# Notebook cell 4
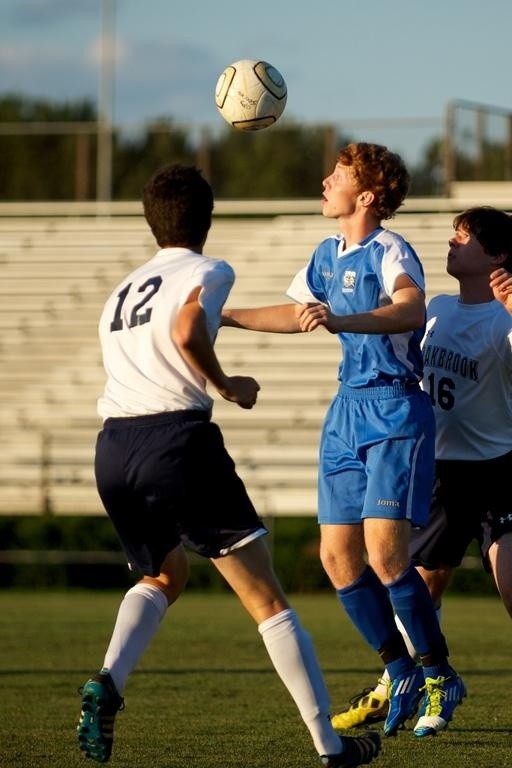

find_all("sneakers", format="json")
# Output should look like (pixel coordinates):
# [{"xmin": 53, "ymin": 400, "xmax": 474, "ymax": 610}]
[
  {"xmin": 321, "ymin": 733, "xmax": 381, "ymax": 768},
  {"xmin": 332, "ymin": 678, "xmax": 390, "ymax": 728},
  {"xmin": 384, "ymin": 667, "xmax": 425, "ymax": 735},
  {"xmin": 413, "ymin": 673, "xmax": 467, "ymax": 736},
  {"xmin": 76, "ymin": 669, "xmax": 123, "ymax": 762}
]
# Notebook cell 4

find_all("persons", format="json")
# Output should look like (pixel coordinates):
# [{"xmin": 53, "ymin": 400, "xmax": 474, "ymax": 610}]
[
  {"xmin": 326, "ymin": 204, "xmax": 512, "ymax": 729},
  {"xmin": 76, "ymin": 163, "xmax": 381, "ymax": 766},
  {"xmin": 213, "ymin": 140, "xmax": 468, "ymax": 737}
]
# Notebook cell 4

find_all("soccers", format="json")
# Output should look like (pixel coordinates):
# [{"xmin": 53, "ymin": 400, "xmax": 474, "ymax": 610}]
[{"xmin": 214, "ymin": 59, "xmax": 286, "ymax": 131}]
